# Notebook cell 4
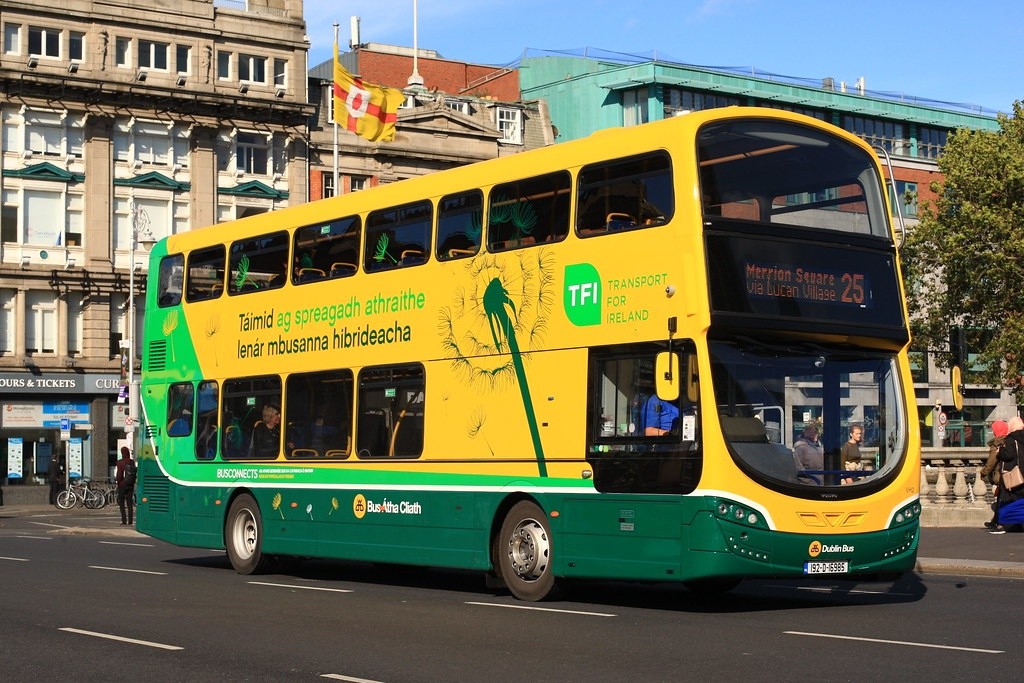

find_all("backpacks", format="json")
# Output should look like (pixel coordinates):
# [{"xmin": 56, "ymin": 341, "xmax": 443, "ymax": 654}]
[{"xmin": 121, "ymin": 459, "xmax": 138, "ymax": 485}]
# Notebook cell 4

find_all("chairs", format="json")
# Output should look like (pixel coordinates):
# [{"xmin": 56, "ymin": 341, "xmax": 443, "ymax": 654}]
[
  {"xmin": 158, "ymin": 213, "xmax": 665, "ymax": 306},
  {"xmin": 628, "ymin": 394, "xmax": 648, "ymax": 451},
  {"xmin": 167, "ymin": 407, "xmax": 417, "ymax": 460}
]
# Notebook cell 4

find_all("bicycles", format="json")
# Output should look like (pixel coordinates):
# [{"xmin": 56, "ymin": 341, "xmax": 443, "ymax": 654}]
[
  {"xmin": 57, "ymin": 477, "xmax": 106, "ymax": 509},
  {"xmin": 80, "ymin": 476, "xmax": 136, "ymax": 510}
]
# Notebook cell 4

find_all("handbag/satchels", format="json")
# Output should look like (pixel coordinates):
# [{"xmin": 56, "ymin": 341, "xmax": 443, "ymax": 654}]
[{"xmin": 1002, "ymin": 466, "xmax": 1024, "ymax": 489}]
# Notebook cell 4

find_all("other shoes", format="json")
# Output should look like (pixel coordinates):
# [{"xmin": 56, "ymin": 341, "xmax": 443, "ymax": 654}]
[
  {"xmin": 990, "ymin": 527, "xmax": 1006, "ymax": 534},
  {"xmin": 985, "ymin": 522, "xmax": 996, "ymax": 529}
]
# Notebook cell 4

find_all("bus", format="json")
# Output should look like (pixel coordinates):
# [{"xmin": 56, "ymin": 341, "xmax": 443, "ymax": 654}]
[{"xmin": 131, "ymin": 104, "xmax": 966, "ymax": 603}]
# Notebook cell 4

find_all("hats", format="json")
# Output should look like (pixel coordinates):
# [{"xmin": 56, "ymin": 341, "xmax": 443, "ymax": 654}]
[
  {"xmin": 1007, "ymin": 417, "xmax": 1024, "ymax": 432},
  {"xmin": 991, "ymin": 421, "xmax": 1009, "ymax": 436}
]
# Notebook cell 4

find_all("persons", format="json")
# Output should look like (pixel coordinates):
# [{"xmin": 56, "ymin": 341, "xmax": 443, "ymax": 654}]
[
  {"xmin": 268, "ymin": 254, "xmax": 303, "ymax": 286},
  {"xmin": 47, "ymin": 454, "xmax": 65, "ymax": 505},
  {"xmin": 254, "ymin": 402, "xmax": 300, "ymax": 460},
  {"xmin": 793, "ymin": 425, "xmax": 827, "ymax": 486},
  {"xmin": 980, "ymin": 417, "xmax": 1024, "ymax": 534},
  {"xmin": 841, "ymin": 424, "xmax": 866, "ymax": 484},
  {"xmin": 114, "ymin": 447, "xmax": 138, "ymax": 526},
  {"xmin": 639, "ymin": 394, "xmax": 678, "ymax": 436}
]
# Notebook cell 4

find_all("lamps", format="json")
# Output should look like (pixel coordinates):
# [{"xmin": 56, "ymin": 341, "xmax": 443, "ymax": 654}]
[
  {"xmin": 274, "ymin": 88, "xmax": 286, "ymax": 100},
  {"xmin": 65, "ymin": 154, "xmax": 75, "ymax": 170},
  {"xmin": 133, "ymin": 262, "xmax": 143, "ymax": 273},
  {"xmin": 66, "ymin": 359, "xmax": 78, "ymax": 367},
  {"xmin": 238, "ymin": 84, "xmax": 250, "ymax": 96},
  {"xmin": 19, "ymin": 256, "xmax": 32, "ymax": 270},
  {"xmin": 172, "ymin": 163, "xmax": 182, "ymax": 180},
  {"xmin": 176, "ymin": 76, "xmax": 186, "ymax": 89},
  {"xmin": 68, "ymin": 63, "xmax": 79, "ymax": 73},
  {"xmin": 64, "ymin": 258, "xmax": 76, "ymax": 271},
  {"xmin": 133, "ymin": 159, "xmax": 143, "ymax": 173},
  {"xmin": 27, "ymin": 58, "xmax": 39, "ymax": 71},
  {"xmin": 234, "ymin": 169, "xmax": 244, "ymax": 184},
  {"xmin": 20, "ymin": 149, "xmax": 33, "ymax": 166},
  {"xmin": 137, "ymin": 72, "xmax": 148, "ymax": 81},
  {"xmin": 272, "ymin": 172, "xmax": 282, "ymax": 188},
  {"xmin": 23, "ymin": 357, "xmax": 34, "ymax": 367}
]
